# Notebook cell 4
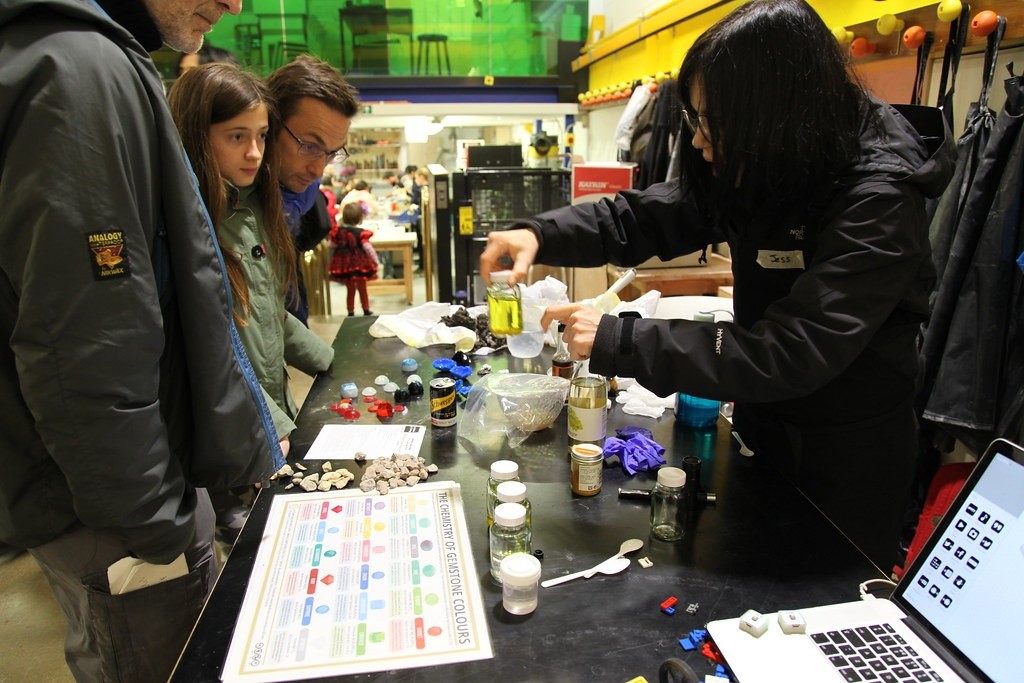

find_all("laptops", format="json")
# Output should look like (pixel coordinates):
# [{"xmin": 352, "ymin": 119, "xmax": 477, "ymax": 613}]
[{"xmin": 703, "ymin": 437, "xmax": 1024, "ymax": 683}]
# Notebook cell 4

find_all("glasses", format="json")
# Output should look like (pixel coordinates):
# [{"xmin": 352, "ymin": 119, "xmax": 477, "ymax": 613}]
[
  {"xmin": 681, "ymin": 109, "xmax": 713, "ymax": 146},
  {"xmin": 282, "ymin": 123, "xmax": 349, "ymax": 165}
]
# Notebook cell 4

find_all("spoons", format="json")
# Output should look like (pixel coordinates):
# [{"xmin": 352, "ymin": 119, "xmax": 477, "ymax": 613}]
[
  {"xmin": 731, "ymin": 431, "xmax": 754, "ymax": 456},
  {"xmin": 584, "ymin": 538, "xmax": 644, "ymax": 579},
  {"xmin": 541, "ymin": 559, "xmax": 631, "ymax": 587}
]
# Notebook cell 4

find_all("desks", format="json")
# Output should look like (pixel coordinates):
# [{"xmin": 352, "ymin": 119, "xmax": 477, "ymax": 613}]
[
  {"xmin": 606, "ymin": 254, "xmax": 734, "ymax": 302},
  {"xmin": 166, "ymin": 316, "xmax": 897, "ymax": 683},
  {"xmin": 365, "ymin": 231, "xmax": 418, "ymax": 307},
  {"xmin": 338, "ymin": 8, "xmax": 414, "ymax": 76}
]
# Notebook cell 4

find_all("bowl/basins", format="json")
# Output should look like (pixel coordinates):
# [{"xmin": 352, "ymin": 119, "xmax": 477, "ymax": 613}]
[
  {"xmin": 489, "ymin": 373, "xmax": 571, "ymax": 432},
  {"xmin": 507, "ymin": 321, "xmax": 544, "ymax": 357}
]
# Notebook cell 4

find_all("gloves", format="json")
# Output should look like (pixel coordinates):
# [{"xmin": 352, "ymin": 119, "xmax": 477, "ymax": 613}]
[{"xmin": 602, "ymin": 426, "xmax": 667, "ymax": 476}]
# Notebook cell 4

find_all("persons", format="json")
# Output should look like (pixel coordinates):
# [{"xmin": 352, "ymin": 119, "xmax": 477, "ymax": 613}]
[
  {"xmin": 479, "ymin": 0, "xmax": 960, "ymax": 581},
  {"xmin": 321, "ymin": 174, "xmax": 338, "ymax": 227},
  {"xmin": 382, "ymin": 164, "xmax": 429, "ymax": 277},
  {"xmin": 341, "ymin": 181, "xmax": 374, "ymax": 206},
  {"xmin": 167, "ymin": 45, "xmax": 358, "ymax": 545},
  {"xmin": 328, "ymin": 203, "xmax": 380, "ymax": 316},
  {"xmin": 0, "ymin": 0, "xmax": 288, "ymax": 683}
]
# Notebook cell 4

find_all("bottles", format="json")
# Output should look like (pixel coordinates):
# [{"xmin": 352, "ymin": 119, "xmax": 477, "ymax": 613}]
[
  {"xmin": 570, "ymin": 444, "xmax": 604, "ymax": 496},
  {"xmin": 650, "ymin": 467, "xmax": 687, "ymax": 543},
  {"xmin": 487, "ymin": 271, "xmax": 522, "ymax": 336},
  {"xmin": 499, "ymin": 552, "xmax": 541, "ymax": 615},
  {"xmin": 567, "ymin": 358, "xmax": 608, "ymax": 454},
  {"xmin": 492, "ymin": 480, "xmax": 532, "ymax": 532},
  {"xmin": 486, "ymin": 460, "xmax": 522, "ymax": 526},
  {"xmin": 552, "ymin": 324, "xmax": 574, "ymax": 410},
  {"xmin": 490, "ymin": 503, "xmax": 532, "ymax": 584},
  {"xmin": 673, "ymin": 309, "xmax": 721, "ymax": 430}
]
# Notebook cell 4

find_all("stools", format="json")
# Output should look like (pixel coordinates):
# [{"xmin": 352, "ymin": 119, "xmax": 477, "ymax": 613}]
[{"xmin": 416, "ymin": 34, "xmax": 451, "ymax": 76}]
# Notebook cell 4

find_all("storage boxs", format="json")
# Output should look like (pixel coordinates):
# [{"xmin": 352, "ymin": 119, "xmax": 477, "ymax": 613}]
[{"xmin": 572, "ymin": 163, "xmax": 640, "ymax": 205}]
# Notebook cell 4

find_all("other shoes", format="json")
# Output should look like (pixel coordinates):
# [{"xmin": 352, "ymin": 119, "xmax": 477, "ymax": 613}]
[
  {"xmin": 349, "ymin": 313, "xmax": 354, "ymax": 316},
  {"xmin": 365, "ymin": 310, "xmax": 372, "ymax": 315}
]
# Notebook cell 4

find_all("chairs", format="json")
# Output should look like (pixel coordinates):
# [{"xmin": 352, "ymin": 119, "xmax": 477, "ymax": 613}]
[{"xmin": 271, "ymin": 15, "xmax": 328, "ymax": 73}]
